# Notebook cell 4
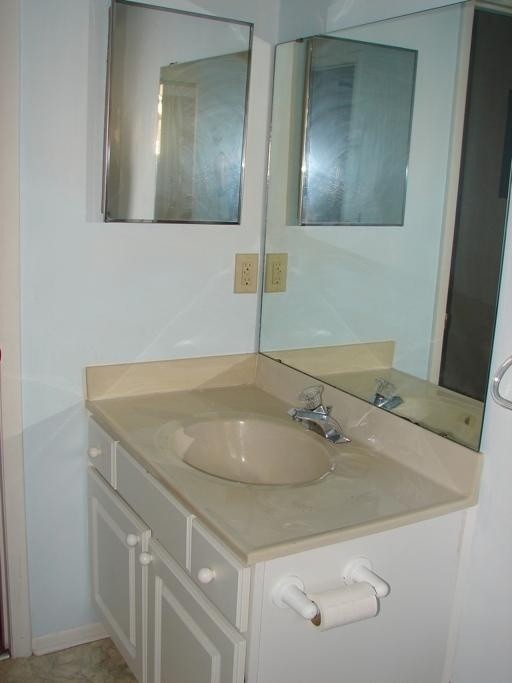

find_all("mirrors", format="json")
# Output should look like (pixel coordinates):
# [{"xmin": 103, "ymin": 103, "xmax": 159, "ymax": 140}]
[
  {"xmin": 96, "ymin": 0, "xmax": 253, "ymax": 228},
  {"xmin": 254, "ymin": 1, "xmax": 511, "ymax": 455},
  {"xmin": 294, "ymin": 32, "xmax": 420, "ymax": 235}
]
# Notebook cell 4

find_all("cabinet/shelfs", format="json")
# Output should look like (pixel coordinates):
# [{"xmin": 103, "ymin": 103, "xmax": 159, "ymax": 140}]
[{"xmin": 86, "ymin": 413, "xmax": 479, "ymax": 682}]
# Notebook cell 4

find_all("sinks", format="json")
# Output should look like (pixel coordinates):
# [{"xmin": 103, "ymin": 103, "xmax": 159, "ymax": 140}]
[
  {"xmin": 382, "ymin": 397, "xmax": 485, "ymax": 451},
  {"xmin": 157, "ymin": 411, "xmax": 338, "ymax": 490}
]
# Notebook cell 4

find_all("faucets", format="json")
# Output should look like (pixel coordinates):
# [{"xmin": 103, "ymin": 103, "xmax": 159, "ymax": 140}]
[
  {"xmin": 373, "ymin": 377, "xmax": 404, "ymax": 411},
  {"xmin": 286, "ymin": 384, "xmax": 350, "ymax": 444}
]
